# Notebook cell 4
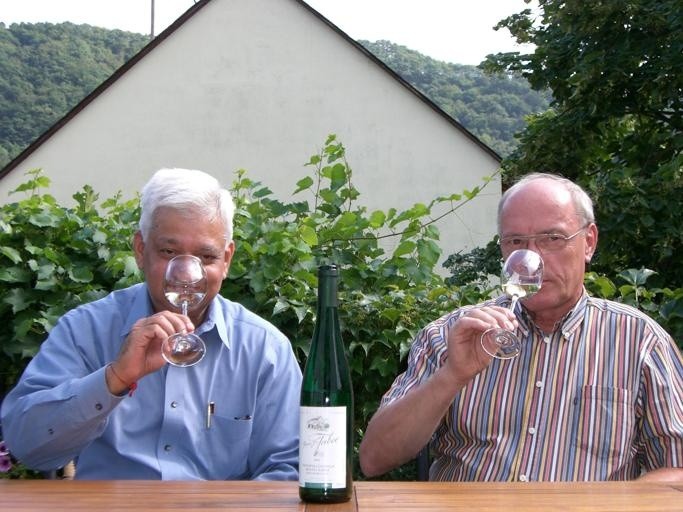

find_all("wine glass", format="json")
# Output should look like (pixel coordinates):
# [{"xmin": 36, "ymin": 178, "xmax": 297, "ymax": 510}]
[
  {"xmin": 479, "ymin": 248, "xmax": 546, "ymax": 359},
  {"xmin": 161, "ymin": 254, "xmax": 206, "ymax": 367}
]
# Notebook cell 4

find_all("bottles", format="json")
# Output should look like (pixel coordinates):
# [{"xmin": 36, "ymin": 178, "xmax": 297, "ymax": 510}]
[{"xmin": 299, "ymin": 264, "xmax": 353, "ymax": 502}]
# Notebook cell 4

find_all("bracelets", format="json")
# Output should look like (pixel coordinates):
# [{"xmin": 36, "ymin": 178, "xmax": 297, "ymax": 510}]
[{"xmin": 109, "ymin": 362, "xmax": 138, "ymax": 396}]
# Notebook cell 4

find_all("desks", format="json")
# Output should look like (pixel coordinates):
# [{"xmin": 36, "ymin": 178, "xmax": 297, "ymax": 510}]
[{"xmin": 1, "ymin": 474, "xmax": 681, "ymax": 508}]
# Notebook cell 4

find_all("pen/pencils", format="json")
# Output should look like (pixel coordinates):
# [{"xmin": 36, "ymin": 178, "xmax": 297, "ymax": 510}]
[{"xmin": 207, "ymin": 402, "xmax": 215, "ymax": 428}]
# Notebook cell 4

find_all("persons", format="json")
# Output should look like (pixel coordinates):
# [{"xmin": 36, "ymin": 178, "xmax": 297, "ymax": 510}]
[
  {"xmin": 1, "ymin": 168, "xmax": 304, "ymax": 482},
  {"xmin": 358, "ymin": 171, "xmax": 683, "ymax": 484}
]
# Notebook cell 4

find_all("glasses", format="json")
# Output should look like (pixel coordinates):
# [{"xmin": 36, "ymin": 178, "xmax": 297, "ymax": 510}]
[{"xmin": 499, "ymin": 227, "xmax": 586, "ymax": 254}]
